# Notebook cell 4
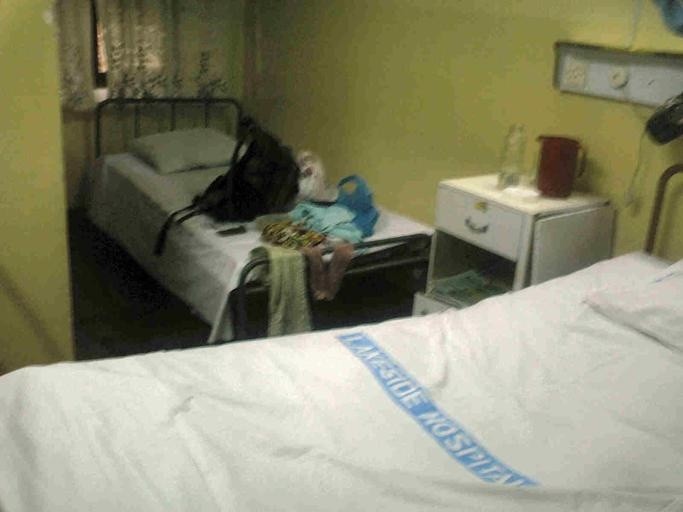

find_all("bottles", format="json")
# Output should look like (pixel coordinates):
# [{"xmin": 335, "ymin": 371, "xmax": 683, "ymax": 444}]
[{"xmin": 495, "ymin": 122, "xmax": 526, "ymax": 194}]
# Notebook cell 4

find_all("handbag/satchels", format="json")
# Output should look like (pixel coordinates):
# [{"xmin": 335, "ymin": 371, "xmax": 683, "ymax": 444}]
[{"xmin": 335, "ymin": 173, "xmax": 381, "ymax": 237}]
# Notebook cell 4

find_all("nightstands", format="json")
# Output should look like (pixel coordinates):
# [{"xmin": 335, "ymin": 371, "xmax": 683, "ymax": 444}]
[{"xmin": 411, "ymin": 173, "xmax": 617, "ymax": 316}]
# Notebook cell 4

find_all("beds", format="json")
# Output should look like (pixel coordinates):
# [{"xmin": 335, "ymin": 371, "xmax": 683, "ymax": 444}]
[
  {"xmin": 0, "ymin": 163, "xmax": 683, "ymax": 512},
  {"xmin": 84, "ymin": 98, "xmax": 436, "ymax": 344}
]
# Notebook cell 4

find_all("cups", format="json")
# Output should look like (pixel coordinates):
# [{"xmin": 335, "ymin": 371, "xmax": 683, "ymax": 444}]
[{"xmin": 531, "ymin": 135, "xmax": 586, "ymax": 198}]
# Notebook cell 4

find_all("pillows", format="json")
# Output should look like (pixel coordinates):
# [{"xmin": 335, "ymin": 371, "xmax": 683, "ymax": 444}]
[
  {"xmin": 588, "ymin": 259, "xmax": 683, "ymax": 354},
  {"xmin": 124, "ymin": 128, "xmax": 249, "ymax": 177}
]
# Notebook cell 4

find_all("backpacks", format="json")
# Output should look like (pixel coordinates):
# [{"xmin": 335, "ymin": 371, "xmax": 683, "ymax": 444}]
[{"xmin": 152, "ymin": 115, "xmax": 300, "ymax": 257}]
